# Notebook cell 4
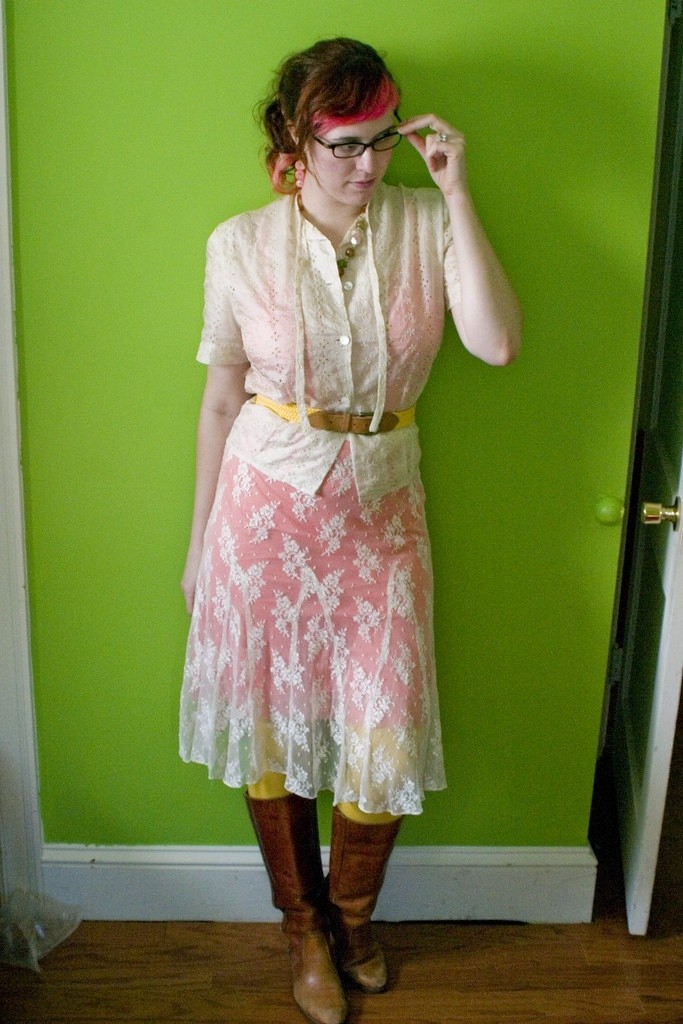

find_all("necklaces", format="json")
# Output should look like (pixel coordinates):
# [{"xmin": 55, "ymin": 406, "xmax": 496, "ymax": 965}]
[{"xmin": 337, "ymin": 205, "xmax": 367, "ymax": 275}]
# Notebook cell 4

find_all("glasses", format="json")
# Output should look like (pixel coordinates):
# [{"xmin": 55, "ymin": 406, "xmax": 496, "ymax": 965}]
[{"xmin": 310, "ymin": 112, "xmax": 403, "ymax": 160}]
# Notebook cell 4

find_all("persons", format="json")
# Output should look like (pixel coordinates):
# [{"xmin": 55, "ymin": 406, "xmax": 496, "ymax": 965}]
[{"xmin": 178, "ymin": 37, "xmax": 520, "ymax": 1024}]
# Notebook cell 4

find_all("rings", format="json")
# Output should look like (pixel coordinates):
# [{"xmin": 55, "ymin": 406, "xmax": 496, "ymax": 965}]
[{"xmin": 440, "ymin": 133, "xmax": 446, "ymax": 142}]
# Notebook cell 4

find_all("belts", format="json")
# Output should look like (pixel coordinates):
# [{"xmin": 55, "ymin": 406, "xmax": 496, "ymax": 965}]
[{"xmin": 255, "ymin": 392, "xmax": 418, "ymax": 436}]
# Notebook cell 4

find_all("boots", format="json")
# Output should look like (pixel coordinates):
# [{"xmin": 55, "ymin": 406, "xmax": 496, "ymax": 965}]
[
  {"xmin": 322, "ymin": 804, "xmax": 405, "ymax": 994},
  {"xmin": 244, "ymin": 787, "xmax": 348, "ymax": 1024}
]
